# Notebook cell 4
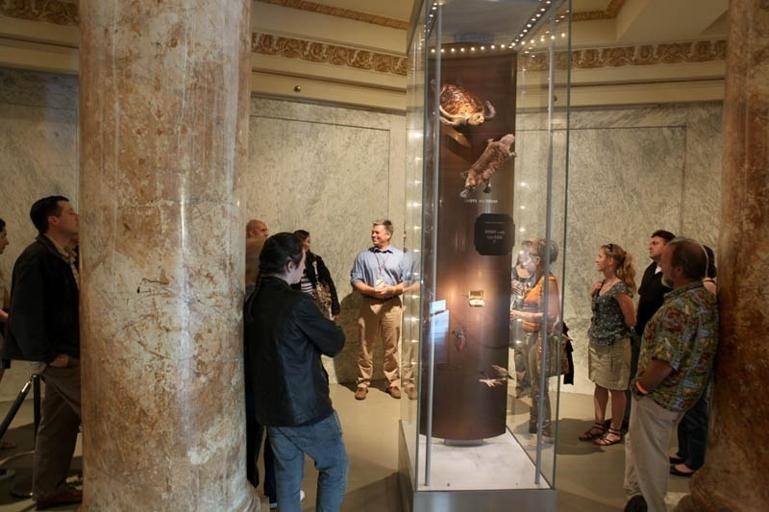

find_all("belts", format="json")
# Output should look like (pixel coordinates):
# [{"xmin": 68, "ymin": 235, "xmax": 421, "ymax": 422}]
[{"xmin": 364, "ymin": 294, "xmax": 398, "ymax": 302}]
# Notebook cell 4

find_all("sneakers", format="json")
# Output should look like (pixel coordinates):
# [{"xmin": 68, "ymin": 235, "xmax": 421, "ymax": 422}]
[
  {"xmin": 623, "ymin": 494, "xmax": 647, "ymax": 511},
  {"xmin": 404, "ymin": 387, "xmax": 418, "ymax": 399},
  {"xmin": 269, "ymin": 490, "xmax": 304, "ymax": 509},
  {"xmin": 387, "ymin": 388, "xmax": 400, "ymax": 398},
  {"xmin": 36, "ymin": 483, "xmax": 82, "ymax": 508},
  {"xmin": 354, "ymin": 387, "xmax": 368, "ymax": 400}
]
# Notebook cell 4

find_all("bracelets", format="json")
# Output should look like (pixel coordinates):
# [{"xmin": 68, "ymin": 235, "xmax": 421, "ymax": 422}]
[{"xmin": 631, "ymin": 380, "xmax": 649, "ymax": 397}]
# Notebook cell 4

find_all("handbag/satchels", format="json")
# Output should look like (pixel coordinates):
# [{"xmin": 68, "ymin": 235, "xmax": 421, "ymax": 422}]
[{"xmin": 536, "ymin": 335, "xmax": 573, "ymax": 377}]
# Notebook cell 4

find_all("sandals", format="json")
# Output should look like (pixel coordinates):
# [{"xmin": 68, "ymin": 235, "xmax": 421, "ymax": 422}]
[
  {"xmin": 594, "ymin": 427, "xmax": 622, "ymax": 445},
  {"xmin": 578, "ymin": 423, "xmax": 607, "ymax": 440}
]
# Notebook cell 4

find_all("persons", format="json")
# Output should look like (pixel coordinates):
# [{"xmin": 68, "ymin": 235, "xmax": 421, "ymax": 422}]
[
  {"xmin": 245, "ymin": 238, "xmax": 307, "ymax": 507},
  {"xmin": 348, "ymin": 217, "xmax": 404, "ymax": 403},
  {"xmin": 600, "ymin": 226, "xmax": 677, "ymax": 432},
  {"xmin": 245, "ymin": 219, "xmax": 271, "ymax": 247},
  {"xmin": 668, "ymin": 245, "xmax": 720, "ymax": 477},
  {"xmin": 7, "ymin": 195, "xmax": 81, "ymax": 509},
  {"xmin": 509, "ymin": 236, "xmax": 569, "ymax": 438},
  {"xmin": 291, "ymin": 230, "xmax": 344, "ymax": 323},
  {"xmin": 403, "ymin": 227, "xmax": 422, "ymax": 400},
  {"xmin": 509, "ymin": 240, "xmax": 533, "ymax": 400},
  {"xmin": 578, "ymin": 241, "xmax": 637, "ymax": 447},
  {"xmin": 0, "ymin": 216, "xmax": 13, "ymax": 383},
  {"xmin": 244, "ymin": 232, "xmax": 352, "ymax": 511},
  {"xmin": 71, "ymin": 231, "xmax": 80, "ymax": 278},
  {"xmin": 620, "ymin": 237, "xmax": 723, "ymax": 512}
]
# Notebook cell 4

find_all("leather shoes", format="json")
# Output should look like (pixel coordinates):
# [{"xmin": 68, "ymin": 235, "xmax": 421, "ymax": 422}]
[
  {"xmin": 670, "ymin": 464, "xmax": 694, "ymax": 476},
  {"xmin": 669, "ymin": 453, "xmax": 685, "ymax": 464}
]
